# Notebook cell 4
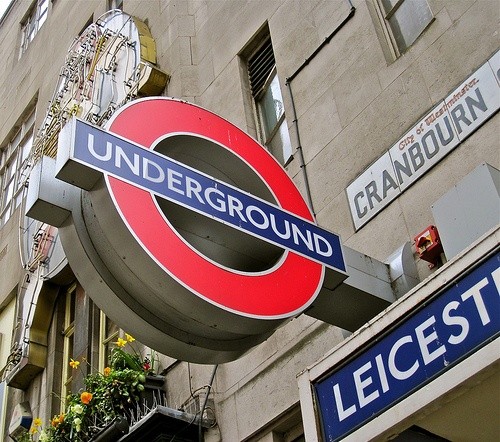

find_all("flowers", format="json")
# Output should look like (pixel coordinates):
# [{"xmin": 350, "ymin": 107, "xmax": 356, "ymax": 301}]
[{"xmin": 27, "ymin": 331, "xmax": 167, "ymax": 442}]
